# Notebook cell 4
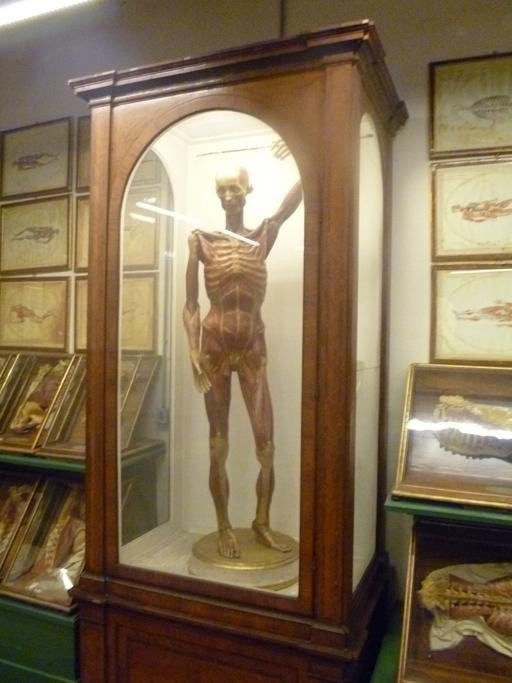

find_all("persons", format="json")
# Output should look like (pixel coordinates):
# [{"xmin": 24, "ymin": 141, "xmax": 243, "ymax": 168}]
[{"xmin": 180, "ymin": 135, "xmax": 304, "ymax": 560}]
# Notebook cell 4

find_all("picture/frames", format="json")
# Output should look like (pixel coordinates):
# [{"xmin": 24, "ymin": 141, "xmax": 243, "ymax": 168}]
[
  {"xmin": 427, "ymin": 50, "xmax": 512, "ymax": 159},
  {"xmin": 75, "ymin": 186, "xmax": 160, "ymax": 273},
  {"xmin": 74, "ymin": 272, "xmax": 158, "ymax": 354},
  {"xmin": 429, "ymin": 263, "xmax": 512, "ymax": 369},
  {"xmin": 0, "ymin": 276, "xmax": 73, "ymax": 353},
  {"xmin": 430, "ymin": 155, "xmax": 512, "ymax": 261},
  {"xmin": 390, "ymin": 363, "xmax": 512, "ymax": 515},
  {"xmin": 76, "ymin": 115, "xmax": 162, "ymax": 193},
  {"xmin": 0, "ymin": 193, "xmax": 73, "ymax": 275},
  {"xmin": 0, "ymin": 116, "xmax": 74, "ymax": 201},
  {"xmin": 0, "ymin": 349, "xmax": 162, "ymax": 615},
  {"xmin": 396, "ymin": 521, "xmax": 512, "ymax": 683}
]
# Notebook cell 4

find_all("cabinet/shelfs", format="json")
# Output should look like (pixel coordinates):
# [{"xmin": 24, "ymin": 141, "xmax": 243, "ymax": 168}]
[
  {"xmin": 370, "ymin": 492, "xmax": 512, "ymax": 683},
  {"xmin": 66, "ymin": 18, "xmax": 408, "ymax": 683},
  {"xmin": 0, "ymin": 439, "xmax": 167, "ymax": 683}
]
